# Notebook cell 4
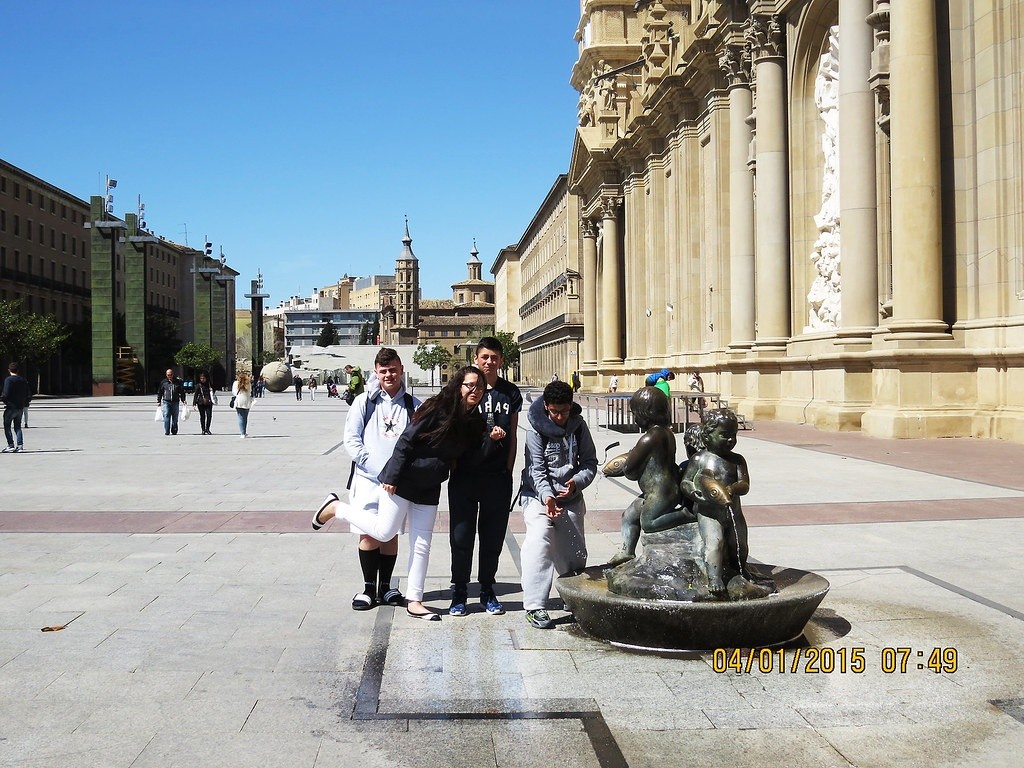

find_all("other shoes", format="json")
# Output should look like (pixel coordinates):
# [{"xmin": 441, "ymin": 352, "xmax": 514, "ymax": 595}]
[
  {"xmin": 312, "ymin": 493, "xmax": 339, "ymax": 531},
  {"xmin": 406, "ymin": 608, "xmax": 440, "ymax": 621},
  {"xmin": 171, "ymin": 428, "xmax": 176, "ymax": 434},
  {"xmin": 14, "ymin": 445, "xmax": 23, "ymax": 452},
  {"xmin": 241, "ymin": 434, "xmax": 245, "ymax": 438},
  {"xmin": 202, "ymin": 430, "xmax": 205, "ymax": 435},
  {"xmin": 2, "ymin": 446, "xmax": 14, "ymax": 453},
  {"xmin": 205, "ymin": 429, "xmax": 210, "ymax": 435},
  {"xmin": 165, "ymin": 430, "xmax": 169, "ymax": 435}
]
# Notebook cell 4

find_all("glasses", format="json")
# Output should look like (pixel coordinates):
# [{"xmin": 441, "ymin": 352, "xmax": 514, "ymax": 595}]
[
  {"xmin": 461, "ymin": 383, "xmax": 486, "ymax": 390},
  {"xmin": 547, "ymin": 407, "xmax": 570, "ymax": 415}
]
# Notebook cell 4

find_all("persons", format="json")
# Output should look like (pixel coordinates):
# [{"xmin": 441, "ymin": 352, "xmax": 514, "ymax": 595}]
[
  {"xmin": 343, "ymin": 347, "xmax": 423, "ymax": 609},
  {"xmin": 519, "ymin": 381, "xmax": 598, "ymax": 627},
  {"xmin": 292, "ymin": 374, "xmax": 303, "ymax": 402},
  {"xmin": 156, "ymin": 369, "xmax": 186, "ymax": 436},
  {"xmin": 344, "ymin": 365, "xmax": 365, "ymax": 398},
  {"xmin": 183, "ymin": 379, "xmax": 194, "ymax": 394},
  {"xmin": 654, "ymin": 377, "xmax": 673, "ymax": 430},
  {"xmin": 308, "ymin": 375, "xmax": 317, "ymax": 401},
  {"xmin": 689, "ymin": 370, "xmax": 704, "ymax": 412},
  {"xmin": 446, "ymin": 336, "xmax": 523, "ymax": 614},
  {"xmin": 311, "ymin": 365, "xmax": 508, "ymax": 620},
  {"xmin": 679, "ymin": 407, "xmax": 750, "ymax": 593},
  {"xmin": 551, "ymin": 372, "xmax": 558, "ymax": 382},
  {"xmin": 326, "ymin": 374, "xmax": 339, "ymax": 398},
  {"xmin": 608, "ymin": 372, "xmax": 618, "ymax": 392},
  {"xmin": 232, "ymin": 373, "xmax": 252, "ymax": 437},
  {"xmin": 608, "ymin": 387, "xmax": 694, "ymax": 567},
  {"xmin": 645, "ymin": 369, "xmax": 675, "ymax": 387},
  {"xmin": 678, "ymin": 423, "xmax": 706, "ymax": 479},
  {"xmin": 0, "ymin": 363, "xmax": 31, "ymax": 453},
  {"xmin": 251, "ymin": 375, "xmax": 266, "ymax": 398},
  {"xmin": 193, "ymin": 373, "xmax": 215, "ymax": 434},
  {"xmin": 572, "ymin": 371, "xmax": 577, "ymax": 392}
]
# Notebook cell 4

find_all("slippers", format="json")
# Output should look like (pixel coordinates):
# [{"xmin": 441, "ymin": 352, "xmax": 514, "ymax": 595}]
[
  {"xmin": 352, "ymin": 593, "xmax": 377, "ymax": 610},
  {"xmin": 377, "ymin": 589, "xmax": 404, "ymax": 606}
]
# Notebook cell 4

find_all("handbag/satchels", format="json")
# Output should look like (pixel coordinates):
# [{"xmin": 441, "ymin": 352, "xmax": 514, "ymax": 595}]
[
  {"xmin": 155, "ymin": 406, "xmax": 164, "ymax": 422},
  {"xmin": 197, "ymin": 383, "xmax": 209, "ymax": 405},
  {"xmin": 606, "ymin": 387, "xmax": 611, "ymax": 393},
  {"xmin": 230, "ymin": 396, "xmax": 237, "ymax": 408},
  {"xmin": 702, "ymin": 399, "xmax": 707, "ymax": 407},
  {"xmin": 180, "ymin": 402, "xmax": 190, "ymax": 422},
  {"xmin": 210, "ymin": 390, "xmax": 217, "ymax": 405}
]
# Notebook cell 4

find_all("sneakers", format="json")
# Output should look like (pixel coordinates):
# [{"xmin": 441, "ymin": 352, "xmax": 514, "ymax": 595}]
[
  {"xmin": 480, "ymin": 589, "xmax": 504, "ymax": 615},
  {"xmin": 449, "ymin": 589, "xmax": 468, "ymax": 616},
  {"xmin": 526, "ymin": 609, "xmax": 554, "ymax": 628}
]
